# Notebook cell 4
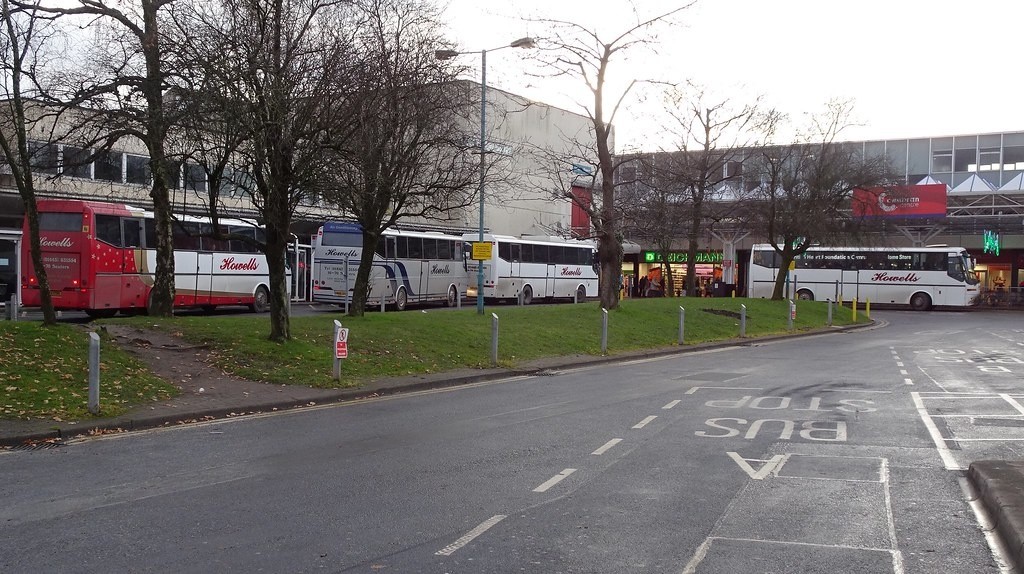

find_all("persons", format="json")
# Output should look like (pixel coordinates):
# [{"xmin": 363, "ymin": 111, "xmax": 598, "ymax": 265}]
[
  {"xmin": 695, "ymin": 277, "xmax": 699, "ymax": 297},
  {"xmin": 704, "ymin": 279, "xmax": 712, "ymax": 297},
  {"xmin": 682, "ymin": 280, "xmax": 687, "ymax": 297},
  {"xmin": 640, "ymin": 275, "xmax": 665, "ymax": 297}
]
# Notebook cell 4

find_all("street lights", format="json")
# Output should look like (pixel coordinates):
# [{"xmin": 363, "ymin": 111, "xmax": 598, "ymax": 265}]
[{"xmin": 435, "ymin": 37, "xmax": 534, "ymax": 313}]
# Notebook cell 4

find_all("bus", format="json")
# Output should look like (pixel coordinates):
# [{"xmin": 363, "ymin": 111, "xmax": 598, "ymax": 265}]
[
  {"xmin": 22, "ymin": 199, "xmax": 293, "ymax": 319},
  {"xmin": 461, "ymin": 234, "xmax": 599, "ymax": 305},
  {"xmin": 746, "ymin": 242, "xmax": 982, "ymax": 311},
  {"xmin": 315, "ymin": 222, "xmax": 473, "ymax": 311}
]
